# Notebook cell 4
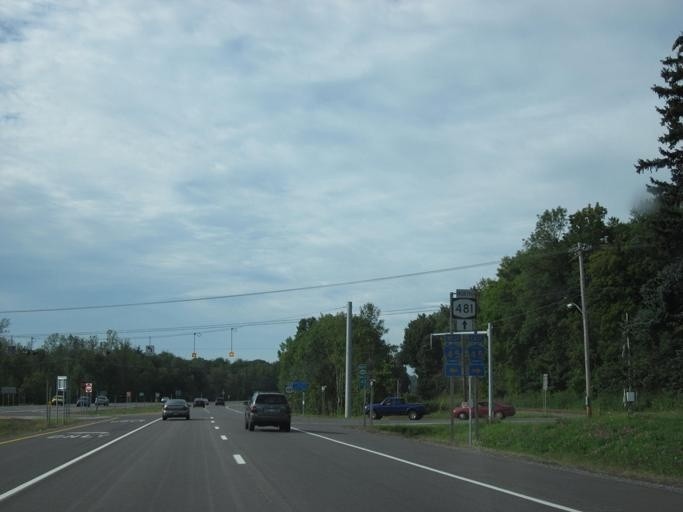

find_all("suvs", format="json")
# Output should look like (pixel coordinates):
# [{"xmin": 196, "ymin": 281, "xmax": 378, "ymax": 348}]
[{"xmin": 240, "ymin": 390, "xmax": 291, "ymax": 432}]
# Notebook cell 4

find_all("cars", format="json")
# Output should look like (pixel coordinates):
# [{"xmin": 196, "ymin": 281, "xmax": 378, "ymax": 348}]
[
  {"xmin": 161, "ymin": 399, "xmax": 191, "ymax": 421},
  {"xmin": 50, "ymin": 396, "xmax": 65, "ymax": 405},
  {"xmin": 94, "ymin": 395, "xmax": 111, "ymax": 406},
  {"xmin": 161, "ymin": 396, "xmax": 169, "ymax": 402},
  {"xmin": 76, "ymin": 396, "xmax": 92, "ymax": 406},
  {"xmin": 452, "ymin": 397, "xmax": 514, "ymax": 421},
  {"xmin": 193, "ymin": 395, "xmax": 225, "ymax": 408}
]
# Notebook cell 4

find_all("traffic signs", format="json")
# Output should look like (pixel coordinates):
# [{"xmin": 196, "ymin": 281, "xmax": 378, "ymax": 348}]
[
  {"xmin": 468, "ymin": 364, "xmax": 485, "ymax": 377},
  {"xmin": 467, "ymin": 334, "xmax": 485, "ymax": 344},
  {"xmin": 358, "ymin": 364, "xmax": 368, "ymax": 385},
  {"xmin": 292, "ymin": 383, "xmax": 310, "ymax": 392},
  {"xmin": 443, "ymin": 333, "xmax": 462, "ymax": 343},
  {"xmin": 444, "ymin": 346, "xmax": 462, "ymax": 363},
  {"xmin": 445, "ymin": 365, "xmax": 462, "ymax": 377},
  {"xmin": 468, "ymin": 345, "xmax": 488, "ymax": 362}
]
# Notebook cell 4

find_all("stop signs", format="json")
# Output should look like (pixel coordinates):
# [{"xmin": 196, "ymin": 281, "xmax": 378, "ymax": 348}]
[{"xmin": 84, "ymin": 383, "xmax": 92, "ymax": 392}]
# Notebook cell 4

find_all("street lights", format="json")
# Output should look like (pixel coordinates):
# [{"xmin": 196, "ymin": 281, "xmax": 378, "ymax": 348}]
[{"xmin": 567, "ymin": 301, "xmax": 591, "ymax": 416}]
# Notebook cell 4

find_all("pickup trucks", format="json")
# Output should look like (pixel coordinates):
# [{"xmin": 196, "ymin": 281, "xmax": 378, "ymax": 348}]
[{"xmin": 362, "ymin": 396, "xmax": 430, "ymax": 421}]
